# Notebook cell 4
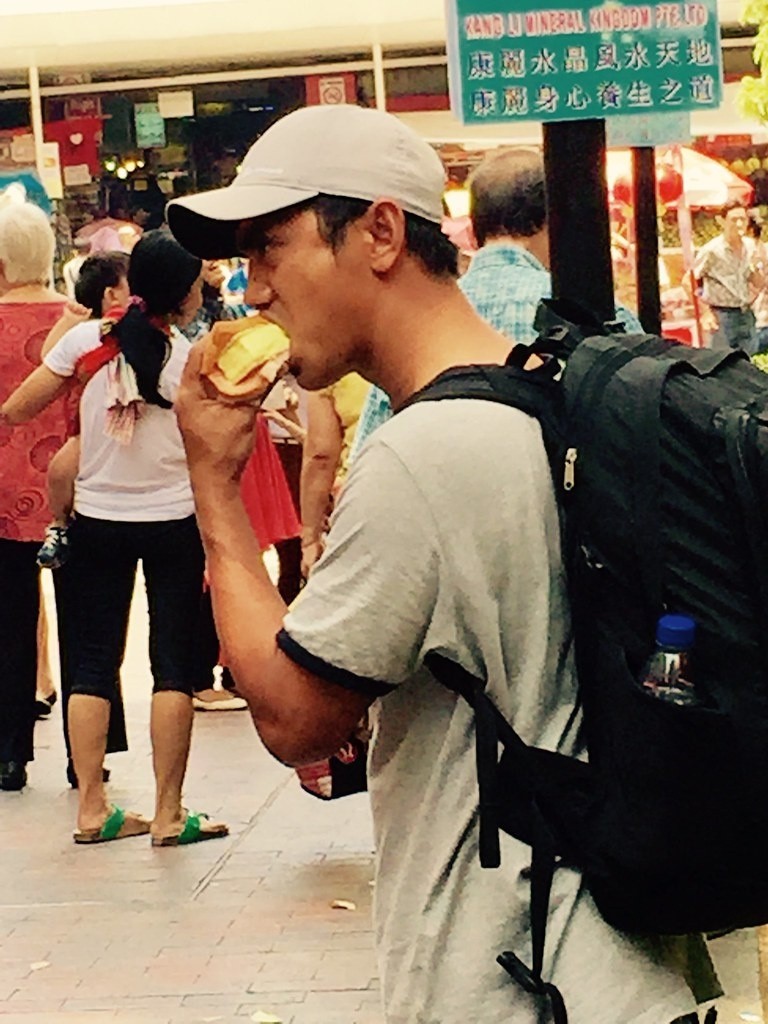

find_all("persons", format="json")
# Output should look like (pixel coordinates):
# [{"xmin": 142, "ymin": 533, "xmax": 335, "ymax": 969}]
[
  {"xmin": 680, "ymin": 199, "xmax": 768, "ymax": 357},
  {"xmin": 161, "ymin": 103, "xmax": 713, "ymax": 1024},
  {"xmin": 0, "ymin": 199, "xmax": 371, "ymax": 849},
  {"xmin": 348, "ymin": 144, "xmax": 647, "ymax": 467}
]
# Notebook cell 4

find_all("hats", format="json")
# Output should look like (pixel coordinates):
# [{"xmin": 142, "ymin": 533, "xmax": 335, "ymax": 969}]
[{"xmin": 164, "ymin": 104, "xmax": 445, "ymax": 260}]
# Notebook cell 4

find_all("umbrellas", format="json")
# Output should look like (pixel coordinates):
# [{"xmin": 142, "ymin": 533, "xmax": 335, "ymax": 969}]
[{"xmin": 604, "ymin": 143, "xmax": 753, "ymax": 348}]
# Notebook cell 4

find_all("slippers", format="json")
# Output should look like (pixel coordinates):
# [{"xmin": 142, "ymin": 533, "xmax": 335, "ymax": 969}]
[
  {"xmin": 150, "ymin": 809, "xmax": 229, "ymax": 847},
  {"xmin": 74, "ymin": 804, "xmax": 150, "ymax": 843}
]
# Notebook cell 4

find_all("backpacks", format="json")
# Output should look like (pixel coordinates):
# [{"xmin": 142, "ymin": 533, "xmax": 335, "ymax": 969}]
[{"xmin": 391, "ymin": 297, "xmax": 768, "ymax": 943}]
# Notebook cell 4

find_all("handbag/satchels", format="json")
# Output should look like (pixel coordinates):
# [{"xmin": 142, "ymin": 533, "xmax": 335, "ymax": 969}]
[{"xmin": 237, "ymin": 410, "xmax": 302, "ymax": 551}]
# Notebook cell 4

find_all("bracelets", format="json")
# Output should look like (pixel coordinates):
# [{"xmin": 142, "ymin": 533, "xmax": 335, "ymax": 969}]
[{"xmin": 299, "ymin": 534, "xmax": 319, "ymax": 546}]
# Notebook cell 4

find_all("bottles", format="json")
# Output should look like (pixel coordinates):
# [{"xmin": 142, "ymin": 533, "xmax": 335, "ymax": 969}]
[{"xmin": 634, "ymin": 615, "xmax": 706, "ymax": 709}]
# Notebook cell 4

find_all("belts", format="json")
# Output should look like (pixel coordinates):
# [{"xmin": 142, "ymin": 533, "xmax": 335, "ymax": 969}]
[{"xmin": 710, "ymin": 305, "xmax": 742, "ymax": 313}]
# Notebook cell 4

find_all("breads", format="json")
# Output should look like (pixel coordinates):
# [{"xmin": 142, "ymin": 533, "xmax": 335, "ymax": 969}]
[{"xmin": 199, "ymin": 310, "xmax": 290, "ymax": 401}]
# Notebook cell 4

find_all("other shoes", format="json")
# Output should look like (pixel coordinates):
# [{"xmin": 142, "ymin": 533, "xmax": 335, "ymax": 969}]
[
  {"xmin": 66, "ymin": 758, "xmax": 109, "ymax": 788},
  {"xmin": 36, "ymin": 517, "xmax": 76, "ymax": 568},
  {"xmin": 35, "ymin": 691, "xmax": 57, "ymax": 720},
  {"xmin": 193, "ymin": 690, "xmax": 249, "ymax": 710},
  {"xmin": 0, "ymin": 762, "xmax": 28, "ymax": 792}
]
